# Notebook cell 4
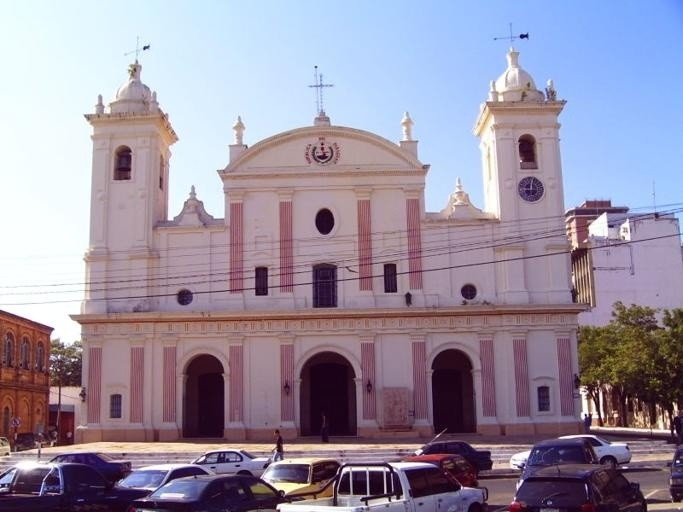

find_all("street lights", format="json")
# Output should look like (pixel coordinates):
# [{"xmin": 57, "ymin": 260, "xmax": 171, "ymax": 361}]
[{"xmin": 54, "ymin": 372, "xmax": 62, "ymax": 431}]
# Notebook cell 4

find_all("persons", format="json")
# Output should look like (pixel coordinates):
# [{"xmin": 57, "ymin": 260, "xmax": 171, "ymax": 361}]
[
  {"xmin": 586, "ymin": 412, "xmax": 593, "ymax": 426},
  {"xmin": 582, "ymin": 414, "xmax": 588, "ymax": 432},
  {"xmin": 670, "ymin": 407, "xmax": 682, "ymax": 445},
  {"xmin": 270, "ymin": 427, "xmax": 284, "ymax": 464},
  {"xmin": 318, "ymin": 411, "xmax": 330, "ymax": 442}
]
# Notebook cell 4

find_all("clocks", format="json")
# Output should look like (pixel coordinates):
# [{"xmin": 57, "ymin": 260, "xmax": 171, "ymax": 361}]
[{"xmin": 518, "ymin": 176, "xmax": 547, "ymax": 204}]
